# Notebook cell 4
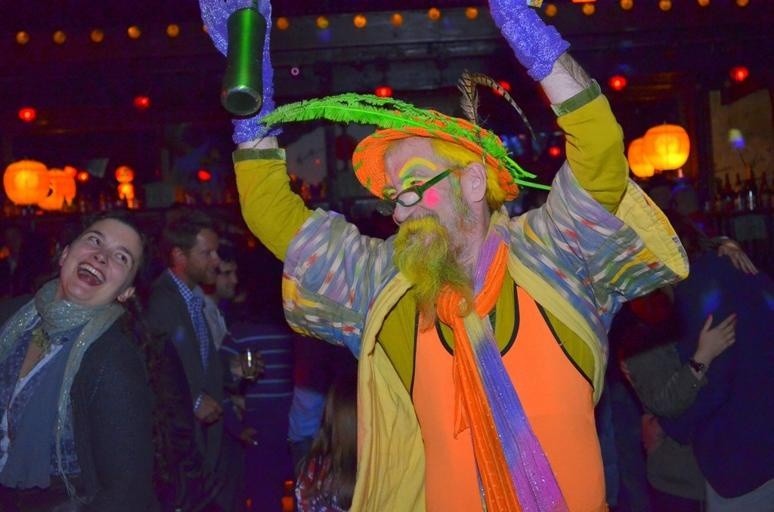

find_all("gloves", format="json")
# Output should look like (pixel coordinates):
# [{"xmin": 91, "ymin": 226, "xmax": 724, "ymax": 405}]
[
  {"xmin": 201, "ymin": 1, "xmax": 284, "ymax": 142},
  {"xmin": 490, "ymin": 0, "xmax": 570, "ymax": 82}
]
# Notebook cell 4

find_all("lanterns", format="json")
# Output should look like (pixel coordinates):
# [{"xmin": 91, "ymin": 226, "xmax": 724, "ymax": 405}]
[
  {"xmin": 1, "ymin": 158, "xmax": 142, "ymax": 212},
  {"xmin": 626, "ymin": 122, "xmax": 691, "ymax": 179}
]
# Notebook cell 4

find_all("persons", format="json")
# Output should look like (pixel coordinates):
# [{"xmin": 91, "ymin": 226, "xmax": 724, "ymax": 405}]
[
  {"xmin": 205, "ymin": 245, "xmax": 240, "ymax": 353},
  {"xmin": 194, "ymin": 0, "xmax": 689, "ymax": 509},
  {"xmin": 602, "ymin": 203, "xmax": 774, "ymax": 511},
  {"xmin": 274, "ymin": 199, "xmax": 398, "ymax": 511},
  {"xmin": 142, "ymin": 214, "xmax": 268, "ymax": 508},
  {"xmin": 1, "ymin": 208, "xmax": 157, "ymax": 511}
]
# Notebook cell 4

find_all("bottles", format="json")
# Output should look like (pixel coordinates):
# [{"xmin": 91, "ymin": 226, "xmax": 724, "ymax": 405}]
[{"xmin": 706, "ymin": 168, "xmax": 773, "ymax": 217}]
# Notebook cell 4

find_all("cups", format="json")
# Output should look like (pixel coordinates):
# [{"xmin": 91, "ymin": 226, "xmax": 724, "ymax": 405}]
[{"xmin": 239, "ymin": 345, "xmax": 258, "ymax": 381}]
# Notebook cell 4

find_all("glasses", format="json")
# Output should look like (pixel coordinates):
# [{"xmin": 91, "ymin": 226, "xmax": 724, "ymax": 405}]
[{"xmin": 375, "ymin": 168, "xmax": 452, "ymax": 216}]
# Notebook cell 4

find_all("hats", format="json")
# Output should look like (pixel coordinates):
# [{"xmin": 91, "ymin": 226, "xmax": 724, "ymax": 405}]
[{"xmin": 353, "ymin": 108, "xmax": 520, "ymax": 203}]
